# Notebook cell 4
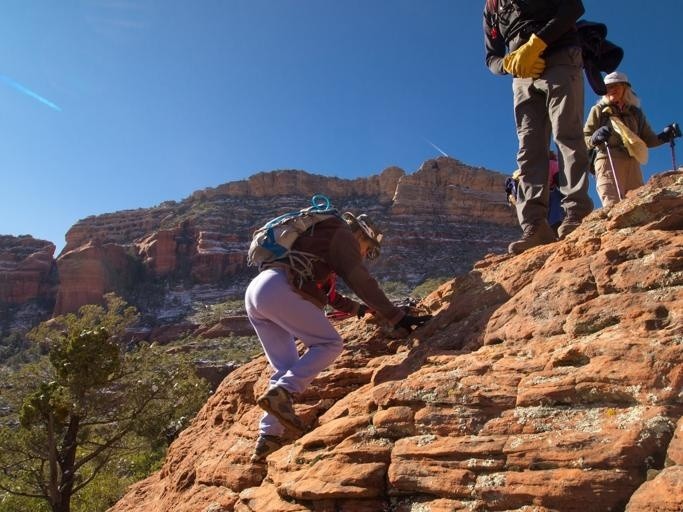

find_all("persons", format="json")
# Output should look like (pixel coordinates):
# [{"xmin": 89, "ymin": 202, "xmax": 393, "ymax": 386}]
[
  {"xmin": 581, "ymin": 71, "xmax": 681, "ymax": 206},
  {"xmin": 482, "ymin": 1, "xmax": 594, "ymax": 255},
  {"xmin": 548, "ymin": 149, "xmax": 565, "ymax": 236},
  {"xmin": 242, "ymin": 210, "xmax": 433, "ymax": 462}
]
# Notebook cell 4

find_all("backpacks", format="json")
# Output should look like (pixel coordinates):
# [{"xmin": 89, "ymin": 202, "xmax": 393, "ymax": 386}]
[{"xmin": 247, "ymin": 195, "xmax": 349, "ymax": 285}]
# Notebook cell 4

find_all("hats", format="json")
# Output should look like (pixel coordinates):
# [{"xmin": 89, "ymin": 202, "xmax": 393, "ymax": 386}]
[
  {"xmin": 341, "ymin": 211, "xmax": 382, "ymax": 263},
  {"xmin": 603, "ymin": 71, "xmax": 631, "ymax": 86}
]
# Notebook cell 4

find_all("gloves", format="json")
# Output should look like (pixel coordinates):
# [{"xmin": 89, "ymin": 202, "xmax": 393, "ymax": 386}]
[
  {"xmin": 591, "ymin": 125, "xmax": 613, "ymax": 146},
  {"xmin": 394, "ymin": 314, "xmax": 433, "ymax": 333},
  {"xmin": 357, "ymin": 303, "xmax": 370, "ymax": 319},
  {"xmin": 663, "ymin": 123, "xmax": 681, "ymax": 142},
  {"xmin": 502, "ymin": 33, "xmax": 547, "ymax": 78}
]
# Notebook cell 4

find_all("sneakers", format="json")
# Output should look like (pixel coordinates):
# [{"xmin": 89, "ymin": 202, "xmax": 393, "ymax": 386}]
[
  {"xmin": 250, "ymin": 436, "xmax": 283, "ymax": 466},
  {"xmin": 257, "ymin": 384, "xmax": 311, "ymax": 433},
  {"xmin": 509, "ymin": 216, "xmax": 556, "ymax": 255},
  {"xmin": 557, "ymin": 209, "xmax": 585, "ymax": 240}
]
